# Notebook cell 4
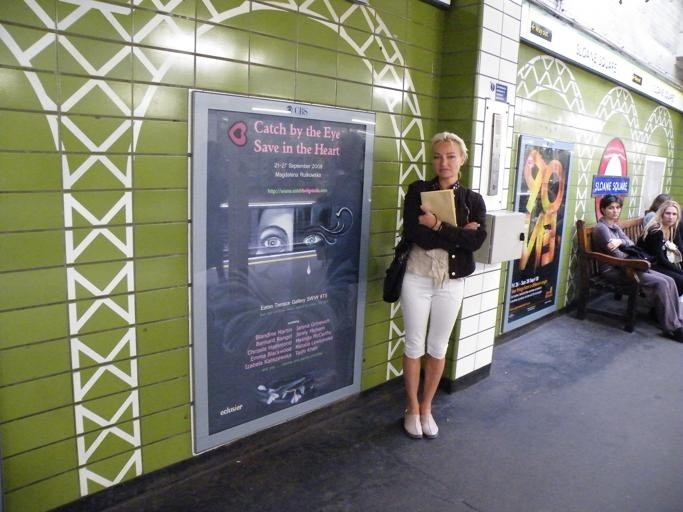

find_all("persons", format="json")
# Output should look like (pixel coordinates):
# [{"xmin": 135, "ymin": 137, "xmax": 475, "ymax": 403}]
[
  {"xmin": 641, "ymin": 193, "xmax": 673, "ymax": 233},
  {"xmin": 253, "ymin": 208, "xmax": 329, "ymax": 253},
  {"xmin": 635, "ymin": 200, "xmax": 683, "ymax": 323},
  {"xmin": 591, "ymin": 196, "xmax": 683, "ymax": 342},
  {"xmin": 398, "ymin": 130, "xmax": 488, "ymax": 438}
]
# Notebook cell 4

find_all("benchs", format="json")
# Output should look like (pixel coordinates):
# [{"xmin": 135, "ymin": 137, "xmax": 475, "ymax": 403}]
[{"xmin": 573, "ymin": 216, "xmax": 652, "ymax": 333}]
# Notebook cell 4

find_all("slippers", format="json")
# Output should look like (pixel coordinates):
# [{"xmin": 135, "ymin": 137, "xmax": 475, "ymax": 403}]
[
  {"xmin": 405, "ymin": 408, "xmax": 424, "ymax": 438},
  {"xmin": 420, "ymin": 413, "xmax": 439, "ymax": 438}
]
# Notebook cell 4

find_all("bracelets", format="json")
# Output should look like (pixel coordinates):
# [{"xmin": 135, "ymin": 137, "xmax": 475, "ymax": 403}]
[{"xmin": 429, "ymin": 214, "xmax": 438, "ymax": 230}]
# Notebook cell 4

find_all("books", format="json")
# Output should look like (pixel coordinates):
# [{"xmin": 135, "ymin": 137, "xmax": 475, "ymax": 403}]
[{"xmin": 420, "ymin": 189, "xmax": 458, "ymax": 228}]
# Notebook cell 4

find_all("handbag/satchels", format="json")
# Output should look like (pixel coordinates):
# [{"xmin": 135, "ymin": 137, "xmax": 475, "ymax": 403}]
[
  {"xmin": 663, "ymin": 226, "xmax": 682, "ymax": 271},
  {"xmin": 382, "ymin": 241, "xmax": 410, "ymax": 303}
]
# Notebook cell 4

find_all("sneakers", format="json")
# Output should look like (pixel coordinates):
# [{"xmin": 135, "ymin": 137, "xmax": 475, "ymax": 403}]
[{"xmin": 664, "ymin": 327, "xmax": 683, "ymax": 342}]
